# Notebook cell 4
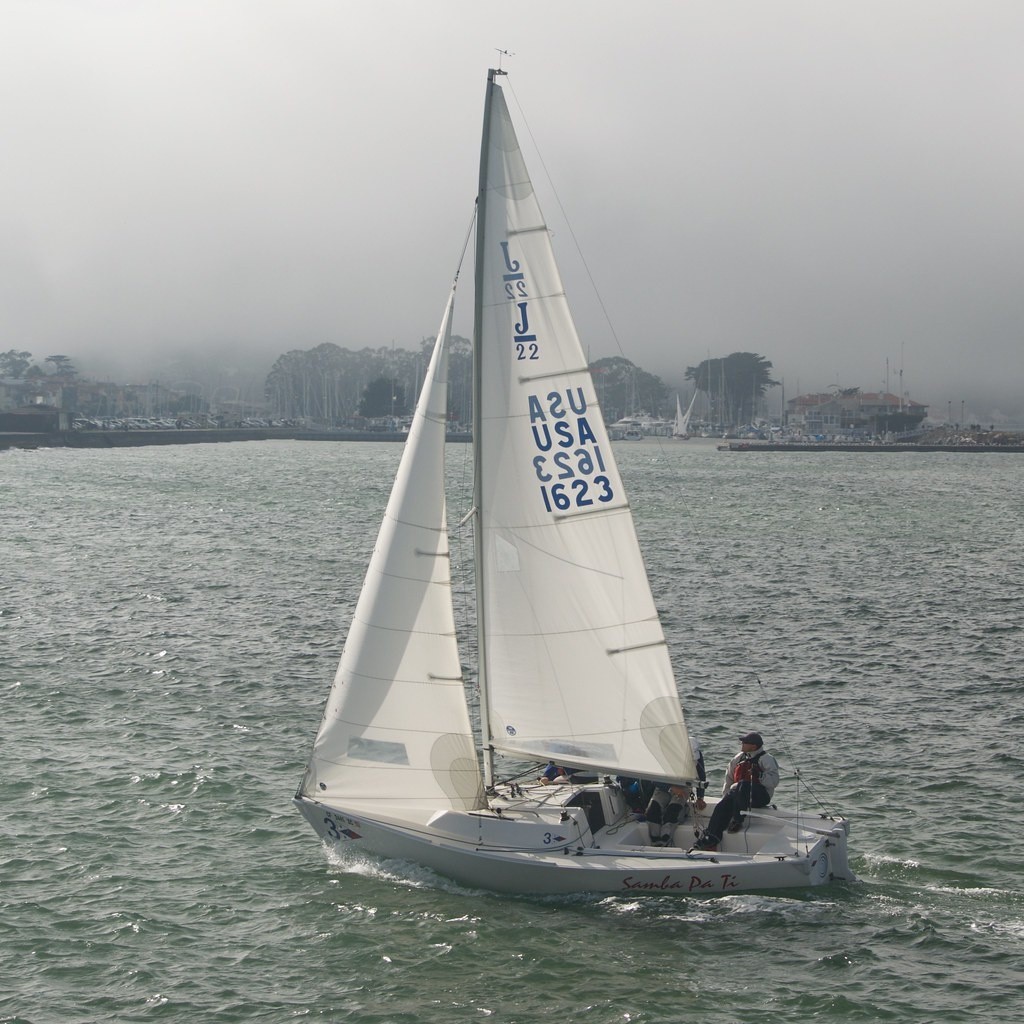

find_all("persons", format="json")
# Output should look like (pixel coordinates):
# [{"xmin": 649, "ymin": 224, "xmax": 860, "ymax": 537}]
[
  {"xmin": 617, "ymin": 775, "xmax": 654, "ymax": 820},
  {"xmin": 647, "ymin": 737, "xmax": 707, "ymax": 846},
  {"xmin": 542, "ymin": 764, "xmax": 598, "ymax": 784},
  {"xmin": 694, "ymin": 732, "xmax": 779, "ymax": 849}
]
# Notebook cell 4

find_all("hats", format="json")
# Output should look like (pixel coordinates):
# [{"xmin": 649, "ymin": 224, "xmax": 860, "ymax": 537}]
[{"xmin": 739, "ymin": 733, "xmax": 764, "ymax": 744}]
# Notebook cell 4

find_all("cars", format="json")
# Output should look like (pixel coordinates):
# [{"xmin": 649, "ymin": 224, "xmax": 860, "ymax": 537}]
[
  {"xmin": 75, "ymin": 418, "xmax": 200, "ymax": 430},
  {"xmin": 239, "ymin": 419, "xmax": 285, "ymax": 428}
]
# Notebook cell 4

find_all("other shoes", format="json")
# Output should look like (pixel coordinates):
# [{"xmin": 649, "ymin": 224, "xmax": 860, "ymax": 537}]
[
  {"xmin": 693, "ymin": 834, "xmax": 719, "ymax": 850},
  {"xmin": 728, "ymin": 817, "xmax": 743, "ymax": 833}
]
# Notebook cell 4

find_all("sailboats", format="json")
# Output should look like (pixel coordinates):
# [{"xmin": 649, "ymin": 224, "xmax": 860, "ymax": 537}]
[{"xmin": 293, "ymin": 45, "xmax": 853, "ymax": 895}]
